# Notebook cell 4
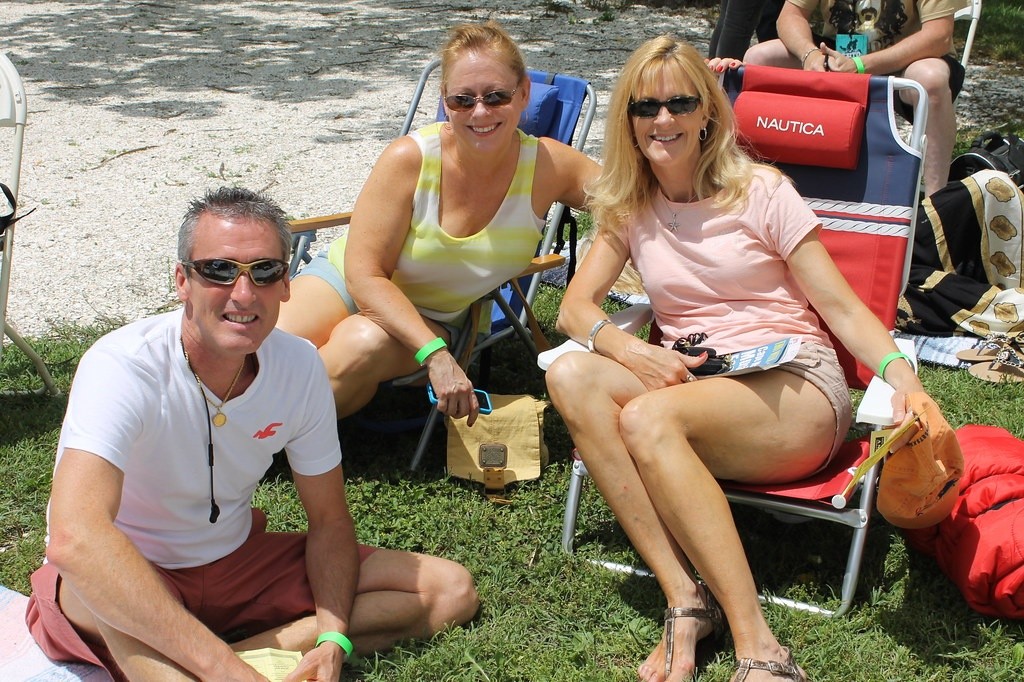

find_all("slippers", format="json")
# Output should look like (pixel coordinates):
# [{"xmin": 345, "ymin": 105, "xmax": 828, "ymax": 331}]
[
  {"xmin": 957, "ymin": 332, "xmax": 1013, "ymax": 361},
  {"xmin": 969, "ymin": 338, "xmax": 1024, "ymax": 383}
]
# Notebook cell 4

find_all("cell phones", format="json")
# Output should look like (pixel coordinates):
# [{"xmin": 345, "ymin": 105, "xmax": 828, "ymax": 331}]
[{"xmin": 426, "ymin": 381, "xmax": 492, "ymax": 415}]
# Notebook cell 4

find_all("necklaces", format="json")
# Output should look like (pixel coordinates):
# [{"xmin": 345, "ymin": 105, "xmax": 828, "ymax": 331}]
[
  {"xmin": 180, "ymin": 337, "xmax": 244, "ymax": 426},
  {"xmin": 660, "ymin": 187, "xmax": 696, "ymax": 231}
]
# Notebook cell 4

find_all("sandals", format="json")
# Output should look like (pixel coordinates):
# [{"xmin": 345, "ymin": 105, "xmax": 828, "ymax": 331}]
[
  {"xmin": 727, "ymin": 644, "xmax": 807, "ymax": 682},
  {"xmin": 640, "ymin": 582, "xmax": 723, "ymax": 682}
]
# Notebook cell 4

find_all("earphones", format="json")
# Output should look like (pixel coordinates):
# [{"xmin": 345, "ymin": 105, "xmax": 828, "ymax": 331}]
[{"xmin": 209, "ymin": 499, "xmax": 220, "ymax": 523}]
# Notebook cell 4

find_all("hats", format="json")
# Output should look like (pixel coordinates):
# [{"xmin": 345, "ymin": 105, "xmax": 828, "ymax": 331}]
[{"xmin": 877, "ymin": 392, "xmax": 962, "ymax": 529}]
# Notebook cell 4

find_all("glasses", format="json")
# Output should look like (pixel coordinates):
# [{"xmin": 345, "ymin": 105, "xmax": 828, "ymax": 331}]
[
  {"xmin": 627, "ymin": 96, "xmax": 701, "ymax": 117},
  {"xmin": 179, "ymin": 257, "xmax": 288, "ymax": 285},
  {"xmin": 445, "ymin": 79, "xmax": 522, "ymax": 113}
]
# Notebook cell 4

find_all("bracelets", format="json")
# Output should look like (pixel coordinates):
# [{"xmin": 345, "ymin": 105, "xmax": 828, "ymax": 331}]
[
  {"xmin": 879, "ymin": 352, "xmax": 915, "ymax": 381},
  {"xmin": 315, "ymin": 631, "xmax": 353, "ymax": 662},
  {"xmin": 414, "ymin": 337, "xmax": 447, "ymax": 367},
  {"xmin": 588, "ymin": 319, "xmax": 612, "ymax": 354},
  {"xmin": 852, "ymin": 56, "xmax": 864, "ymax": 74},
  {"xmin": 801, "ymin": 46, "xmax": 821, "ymax": 67}
]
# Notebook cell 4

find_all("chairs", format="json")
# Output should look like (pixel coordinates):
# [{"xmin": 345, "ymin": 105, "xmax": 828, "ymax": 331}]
[
  {"xmin": 285, "ymin": 57, "xmax": 597, "ymax": 472},
  {"xmin": 563, "ymin": 65, "xmax": 928, "ymax": 618},
  {"xmin": 953, "ymin": 0, "xmax": 982, "ymax": 70},
  {"xmin": 0, "ymin": 52, "xmax": 62, "ymax": 396}
]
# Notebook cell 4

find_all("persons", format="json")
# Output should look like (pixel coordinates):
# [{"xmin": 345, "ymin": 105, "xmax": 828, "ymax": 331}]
[
  {"xmin": 275, "ymin": 18, "xmax": 601, "ymax": 424},
  {"xmin": 24, "ymin": 177, "xmax": 477, "ymax": 681},
  {"xmin": 544, "ymin": 36, "xmax": 928, "ymax": 682},
  {"xmin": 743, "ymin": 0, "xmax": 965, "ymax": 199}
]
